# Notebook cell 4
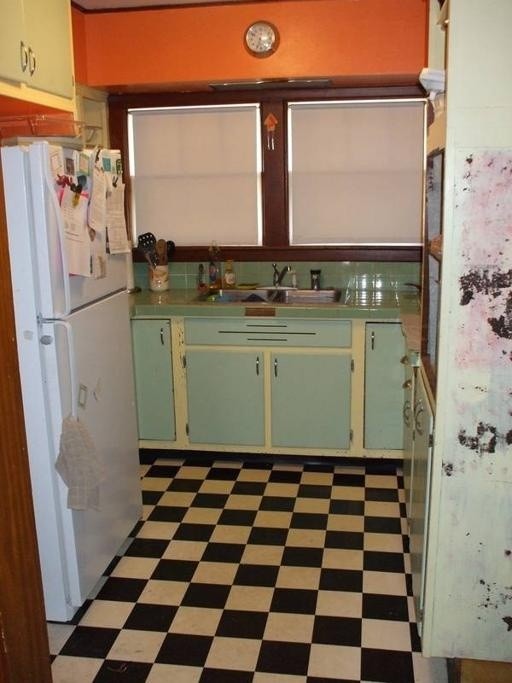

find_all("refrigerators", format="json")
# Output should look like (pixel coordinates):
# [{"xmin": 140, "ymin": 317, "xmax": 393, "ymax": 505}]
[{"xmin": 1, "ymin": 141, "xmax": 144, "ymax": 626}]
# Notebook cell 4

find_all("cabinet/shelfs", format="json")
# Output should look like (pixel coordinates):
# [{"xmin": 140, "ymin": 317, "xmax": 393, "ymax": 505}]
[
  {"xmin": 364, "ymin": 308, "xmax": 405, "ymax": 466},
  {"xmin": 402, "ymin": 340, "xmax": 436, "ymax": 637},
  {"xmin": 130, "ymin": 305, "xmax": 176, "ymax": 457},
  {"xmin": 0, "ymin": 0, "xmax": 76, "ymax": 113},
  {"xmin": 184, "ymin": 305, "xmax": 354, "ymax": 463},
  {"xmin": 0, "ymin": 84, "xmax": 111, "ymax": 150}
]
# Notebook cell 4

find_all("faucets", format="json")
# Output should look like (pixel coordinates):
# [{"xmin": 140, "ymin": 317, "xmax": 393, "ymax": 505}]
[{"xmin": 272, "ymin": 263, "xmax": 292, "ymax": 286}]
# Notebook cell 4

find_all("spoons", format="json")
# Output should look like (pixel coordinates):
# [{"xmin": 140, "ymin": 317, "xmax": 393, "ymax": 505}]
[{"xmin": 156, "ymin": 239, "xmax": 175, "ymax": 265}]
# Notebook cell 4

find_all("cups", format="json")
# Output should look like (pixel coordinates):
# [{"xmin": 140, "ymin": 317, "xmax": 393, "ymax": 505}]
[
  {"xmin": 356, "ymin": 272, "xmax": 385, "ymax": 291},
  {"xmin": 148, "ymin": 265, "xmax": 169, "ymax": 293}
]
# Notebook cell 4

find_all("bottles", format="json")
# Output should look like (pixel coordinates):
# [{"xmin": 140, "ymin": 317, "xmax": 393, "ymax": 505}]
[
  {"xmin": 311, "ymin": 270, "xmax": 321, "ymax": 289},
  {"xmin": 197, "ymin": 256, "xmax": 236, "ymax": 295}
]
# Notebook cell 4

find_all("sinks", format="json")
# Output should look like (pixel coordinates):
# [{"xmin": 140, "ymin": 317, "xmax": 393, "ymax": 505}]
[
  {"xmin": 193, "ymin": 286, "xmax": 273, "ymax": 304},
  {"xmin": 273, "ymin": 288, "xmax": 342, "ymax": 303}
]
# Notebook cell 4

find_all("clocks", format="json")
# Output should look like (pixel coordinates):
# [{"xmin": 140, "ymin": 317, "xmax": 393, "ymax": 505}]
[{"xmin": 243, "ymin": 21, "xmax": 280, "ymax": 59}]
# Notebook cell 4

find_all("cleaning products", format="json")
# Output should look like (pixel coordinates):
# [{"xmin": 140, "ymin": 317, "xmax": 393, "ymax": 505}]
[
  {"xmin": 310, "ymin": 268, "xmax": 321, "ymax": 289},
  {"xmin": 224, "ymin": 259, "xmax": 236, "ymax": 288},
  {"xmin": 288, "ymin": 269, "xmax": 298, "ymax": 287}
]
